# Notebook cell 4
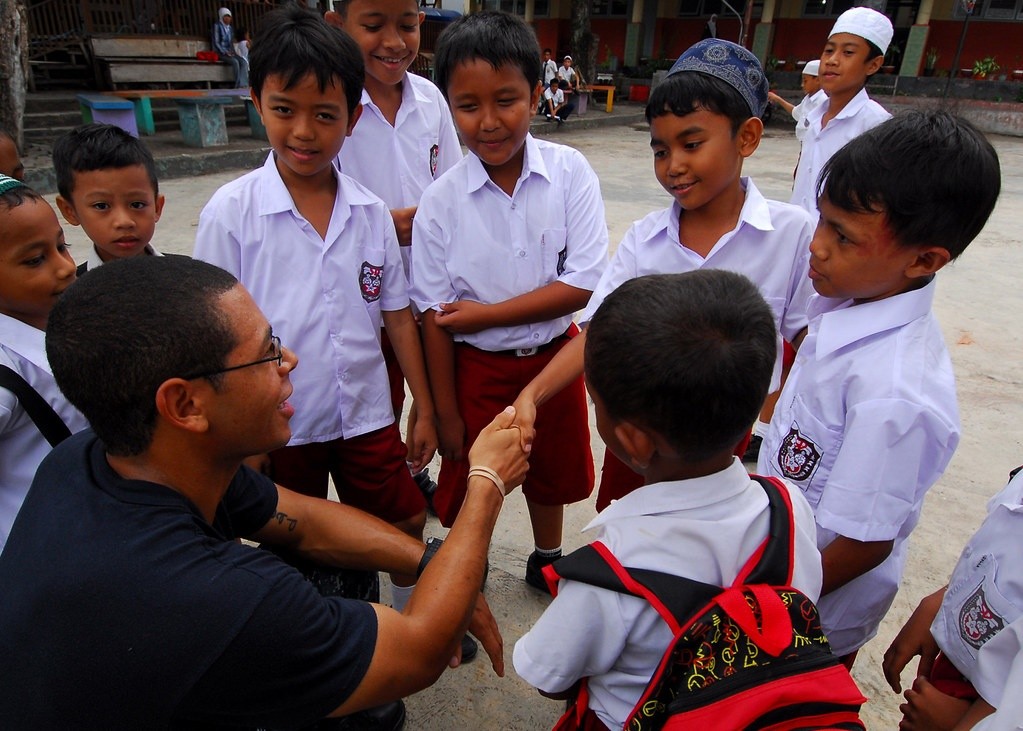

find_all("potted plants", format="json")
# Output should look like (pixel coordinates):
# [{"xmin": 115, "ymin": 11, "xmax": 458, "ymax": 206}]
[
  {"xmin": 923, "ymin": 46, "xmax": 938, "ymax": 76},
  {"xmin": 972, "ymin": 58, "xmax": 999, "ymax": 79},
  {"xmin": 882, "ymin": 44, "xmax": 900, "ymax": 74},
  {"xmin": 784, "ymin": 55, "xmax": 799, "ymax": 70}
]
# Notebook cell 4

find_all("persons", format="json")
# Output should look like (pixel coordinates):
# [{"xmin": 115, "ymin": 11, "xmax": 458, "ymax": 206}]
[
  {"xmin": 0, "ymin": 127, "xmax": 25, "ymax": 184},
  {"xmin": 0, "ymin": 174, "xmax": 91, "ymax": 556},
  {"xmin": 507, "ymin": 7, "xmax": 1023, "ymax": 731},
  {"xmin": 51, "ymin": 121, "xmax": 166, "ymax": 277},
  {"xmin": 192, "ymin": 3, "xmax": 477, "ymax": 665},
  {"xmin": 0, "ymin": 254, "xmax": 535, "ymax": 731},
  {"xmin": 407, "ymin": 10, "xmax": 610, "ymax": 597},
  {"xmin": 325, "ymin": 0, "xmax": 465, "ymax": 515}
]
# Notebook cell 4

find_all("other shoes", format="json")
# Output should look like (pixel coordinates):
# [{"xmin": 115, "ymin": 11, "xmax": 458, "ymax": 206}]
[
  {"xmin": 742, "ymin": 434, "xmax": 763, "ymax": 463},
  {"xmin": 414, "ymin": 468, "xmax": 437, "ymax": 516},
  {"xmin": 526, "ymin": 551, "xmax": 559, "ymax": 594},
  {"xmin": 461, "ymin": 634, "xmax": 477, "ymax": 664}
]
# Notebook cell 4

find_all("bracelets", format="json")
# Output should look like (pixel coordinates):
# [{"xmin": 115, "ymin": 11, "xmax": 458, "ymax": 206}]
[
  {"xmin": 416, "ymin": 536, "xmax": 446, "ymax": 579},
  {"xmin": 465, "ymin": 467, "xmax": 508, "ymax": 499}
]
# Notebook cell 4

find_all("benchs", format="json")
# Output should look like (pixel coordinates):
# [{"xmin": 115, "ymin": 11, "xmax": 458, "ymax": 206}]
[
  {"xmin": 543, "ymin": 83, "xmax": 617, "ymax": 115},
  {"xmin": 76, "ymin": 35, "xmax": 271, "ymax": 144}
]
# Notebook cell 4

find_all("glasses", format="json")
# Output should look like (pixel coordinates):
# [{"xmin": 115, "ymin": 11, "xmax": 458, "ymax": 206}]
[{"xmin": 185, "ymin": 336, "xmax": 282, "ymax": 379}]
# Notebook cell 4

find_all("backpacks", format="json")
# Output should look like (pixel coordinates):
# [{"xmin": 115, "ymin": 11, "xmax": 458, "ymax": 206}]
[{"xmin": 555, "ymin": 474, "xmax": 872, "ymax": 731}]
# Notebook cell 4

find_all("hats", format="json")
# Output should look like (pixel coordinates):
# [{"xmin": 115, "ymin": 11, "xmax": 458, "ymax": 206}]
[
  {"xmin": 564, "ymin": 55, "xmax": 573, "ymax": 61},
  {"xmin": 0, "ymin": 173, "xmax": 22, "ymax": 196},
  {"xmin": 827, "ymin": 6, "xmax": 894, "ymax": 56},
  {"xmin": 802, "ymin": 60, "xmax": 821, "ymax": 76},
  {"xmin": 664, "ymin": 38, "xmax": 769, "ymax": 119}
]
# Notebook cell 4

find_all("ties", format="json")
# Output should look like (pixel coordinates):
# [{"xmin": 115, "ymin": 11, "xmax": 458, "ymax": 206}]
[{"xmin": 542, "ymin": 64, "xmax": 547, "ymax": 86}]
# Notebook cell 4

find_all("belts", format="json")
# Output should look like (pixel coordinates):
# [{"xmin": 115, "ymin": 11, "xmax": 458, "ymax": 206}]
[{"xmin": 496, "ymin": 334, "xmax": 567, "ymax": 357}]
